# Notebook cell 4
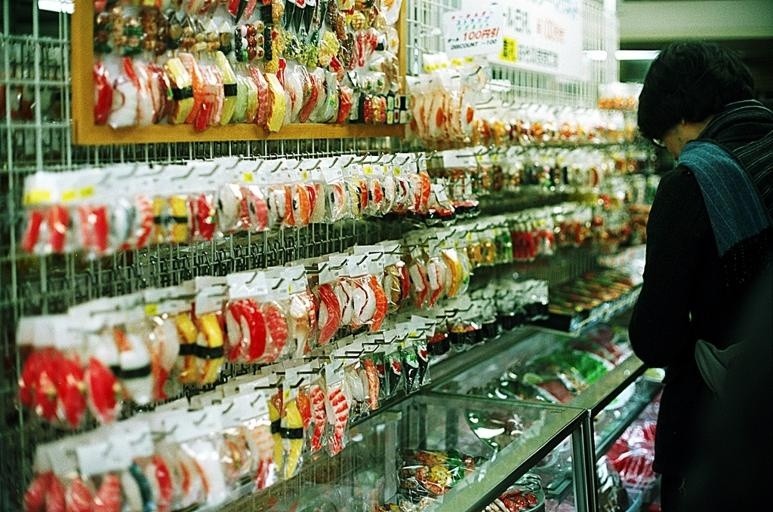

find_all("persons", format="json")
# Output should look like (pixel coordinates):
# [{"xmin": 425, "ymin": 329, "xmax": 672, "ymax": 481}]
[{"xmin": 627, "ymin": 39, "xmax": 773, "ymax": 512}]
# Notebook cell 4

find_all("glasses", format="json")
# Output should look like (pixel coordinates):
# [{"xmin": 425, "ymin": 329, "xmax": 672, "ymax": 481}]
[{"xmin": 648, "ymin": 121, "xmax": 678, "ymax": 149}]
